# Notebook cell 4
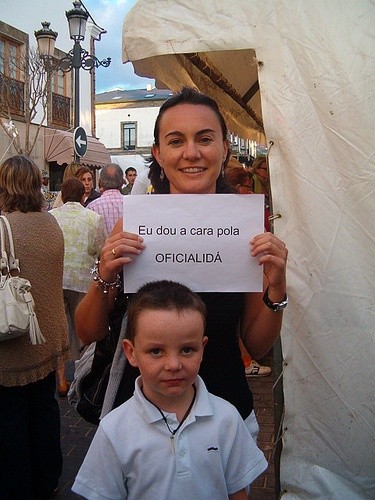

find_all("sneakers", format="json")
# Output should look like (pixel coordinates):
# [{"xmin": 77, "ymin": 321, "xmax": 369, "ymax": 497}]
[{"xmin": 244, "ymin": 360, "xmax": 272, "ymax": 377}]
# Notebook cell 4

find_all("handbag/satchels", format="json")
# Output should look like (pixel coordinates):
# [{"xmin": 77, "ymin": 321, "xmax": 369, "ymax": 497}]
[
  {"xmin": 68, "ymin": 287, "xmax": 128, "ymax": 426},
  {"xmin": 0, "ymin": 215, "xmax": 46, "ymax": 345}
]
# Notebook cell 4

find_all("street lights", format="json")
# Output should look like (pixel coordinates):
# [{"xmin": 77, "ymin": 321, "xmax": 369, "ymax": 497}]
[{"xmin": 34, "ymin": 0, "xmax": 111, "ymax": 163}]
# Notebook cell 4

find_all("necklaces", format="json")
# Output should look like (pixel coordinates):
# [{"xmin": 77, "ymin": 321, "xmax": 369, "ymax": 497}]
[{"xmin": 140, "ymin": 383, "xmax": 197, "ymax": 453}]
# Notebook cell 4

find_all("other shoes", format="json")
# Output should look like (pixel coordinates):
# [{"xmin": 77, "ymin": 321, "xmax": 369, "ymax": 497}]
[{"xmin": 58, "ymin": 381, "xmax": 70, "ymax": 396}]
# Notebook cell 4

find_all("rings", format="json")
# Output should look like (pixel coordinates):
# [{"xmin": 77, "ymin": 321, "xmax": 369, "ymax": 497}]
[{"xmin": 112, "ymin": 249, "xmax": 116, "ymax": 254}]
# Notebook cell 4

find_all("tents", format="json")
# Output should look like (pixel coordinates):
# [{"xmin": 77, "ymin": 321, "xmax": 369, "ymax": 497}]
[{"xmin": 121, "ymin": 0, "xmax": 375, "ymax": 500}]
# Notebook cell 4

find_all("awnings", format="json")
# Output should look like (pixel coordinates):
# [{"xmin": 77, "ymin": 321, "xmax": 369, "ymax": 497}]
[{"xmin": 44, "ymin": 128, "xmax": 111, "ymax": 171}]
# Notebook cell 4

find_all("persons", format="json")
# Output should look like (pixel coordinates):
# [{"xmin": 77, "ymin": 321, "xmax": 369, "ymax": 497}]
[
  {"xmin": 0, "ymin": 157, "xmax": 272, "ymax": 500},
  {"xmin": 66, "ymin": 88, "xmax": 289, "ymax": 497}
]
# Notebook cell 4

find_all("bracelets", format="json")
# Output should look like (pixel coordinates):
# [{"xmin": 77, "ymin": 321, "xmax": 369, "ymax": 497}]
[{"xmin": 89, "ymin": 260, "xmax": 122, "ymax": 294}]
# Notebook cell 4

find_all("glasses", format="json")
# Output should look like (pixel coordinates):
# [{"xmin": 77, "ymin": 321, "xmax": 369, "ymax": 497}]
[{"xmin": 260, "ymin": 167, "xmax": 267, "ymax": 171}]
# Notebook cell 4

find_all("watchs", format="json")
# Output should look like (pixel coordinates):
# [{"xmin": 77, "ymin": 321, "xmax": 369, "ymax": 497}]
[{"xmin": 262, "ymin": 285, "xmax": 288, "ymax": 313}]
